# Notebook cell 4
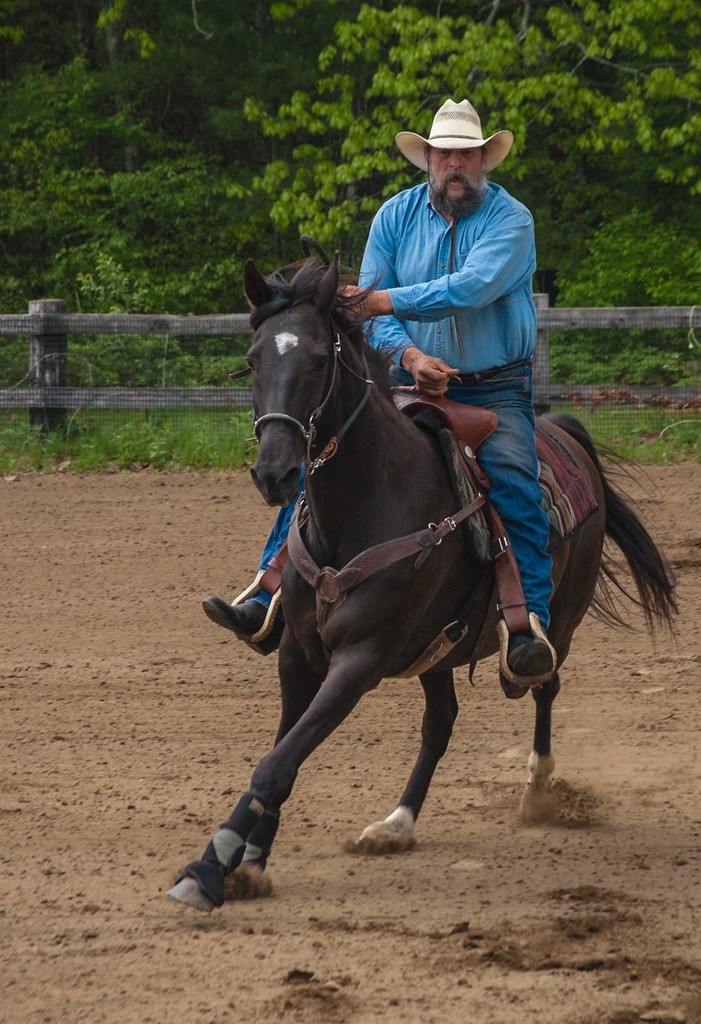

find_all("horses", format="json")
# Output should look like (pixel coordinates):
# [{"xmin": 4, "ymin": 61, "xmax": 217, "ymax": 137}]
[{"xmin": 165, "ymin": 250, "xmax": 686, "ymax": 913}]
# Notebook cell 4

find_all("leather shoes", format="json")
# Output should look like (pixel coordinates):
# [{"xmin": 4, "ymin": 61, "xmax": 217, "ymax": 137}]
[
  {"xmin": 202, "ymin": 597, "xmax": 285, "ymax": 657},
  {"xmin": 500, "ymin": 630, "xmax": 553, "ymax": 699}
]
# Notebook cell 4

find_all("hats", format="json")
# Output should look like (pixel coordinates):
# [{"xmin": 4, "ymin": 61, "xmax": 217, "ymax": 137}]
[{"xmin": 395, "ymin": 99, "xmax": 514, "ymax": 174}]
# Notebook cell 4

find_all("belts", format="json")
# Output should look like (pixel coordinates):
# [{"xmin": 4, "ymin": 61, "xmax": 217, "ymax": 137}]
[{"xmin": 448, "ymin": 359, "xmax": 527, "ymax": 387}]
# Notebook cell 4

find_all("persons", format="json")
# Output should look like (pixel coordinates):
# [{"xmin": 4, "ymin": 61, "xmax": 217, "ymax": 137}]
[{"xmin": 202, "ymin": 100, "xmax": 554, "ymax": 700}]
